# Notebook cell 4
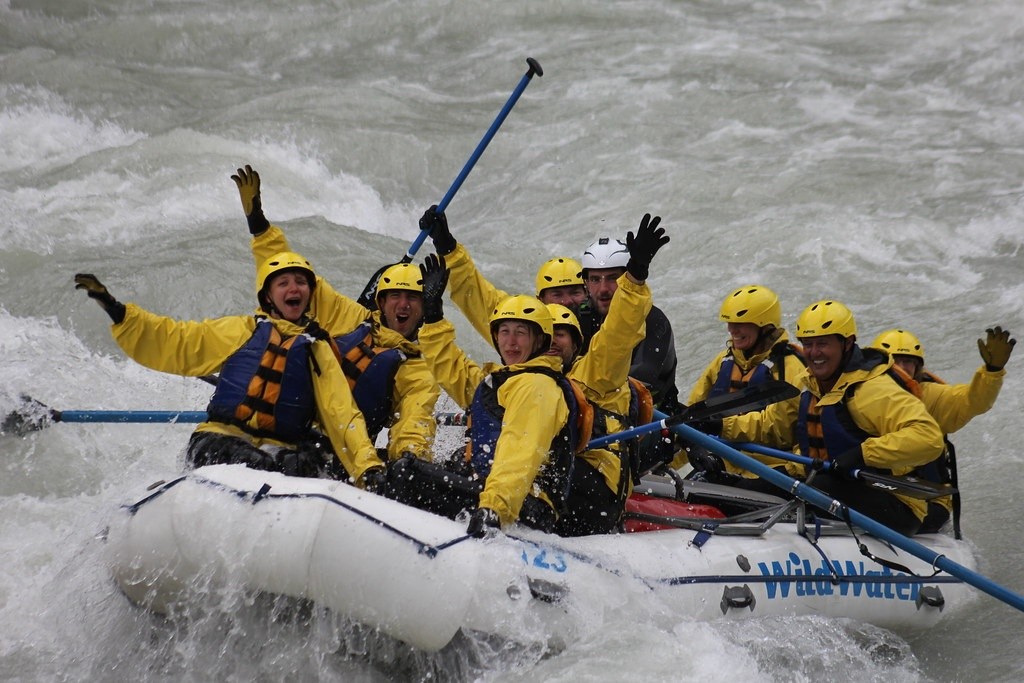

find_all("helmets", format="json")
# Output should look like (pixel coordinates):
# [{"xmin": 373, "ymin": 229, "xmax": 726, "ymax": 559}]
[
  {"xmin": 794, "ymin": 299, "xmax": 858, "ymax": 346},
  {"xmin": 375, "ymin": 263, "xmax": 424, "ymax": 311},
  {"xmin": 535, "ymin": 256, "xmax": 585, "ymax": 298},
  {"xmin": 255, "ymin": 252, "xmax": 318, "ymax": 315},
  {"xmin": 544, "ymin": 303, "xmax": 584, "ymax": 357},
  {"xmin": 488, "ymin": 294, "xmax": 552, "ymax": 357},
  {"xmin": 581, "ymin": 237, "xmax": 630, "ymax": 278},
  {"xmin": 719, "ymin": 285, "xmax": 781, "ymax": 332},
  {"xmin": 870, "ymin": 328, "xmax": 925, "ymax": 369}
]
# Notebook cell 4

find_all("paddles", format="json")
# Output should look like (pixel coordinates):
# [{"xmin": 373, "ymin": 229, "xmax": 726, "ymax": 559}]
[
  {"xmin": 2, "ymin": 393, "xmax": 211, "ymax": 426},
  {"xmin": 358, "ymin": 55, "xmax": 546, "ymax": 309},
  {"xmin": 649, "ymin": 406, "xmax": 1022, "ymax": 613},
  {"xmin": 715, "ymin": 434, "xmax": 957, "ymax": 501},
  {"xmin": 587, "ymin": 389, "xmax": 748, "ymax": 450}
]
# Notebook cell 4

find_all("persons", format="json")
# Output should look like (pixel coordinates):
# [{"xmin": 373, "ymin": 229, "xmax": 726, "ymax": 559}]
[
  {"xmin": 418, "ymin": 205, "xmax": 585, "ymax": 349},
  {"xmin": 74, "ymin": 251, "xmax": 387, "ymax": 496},
  {"xmin": 687, "ymin": 284, "xmax": 807, "ymax": 494},
  {"xmin": 566, "ymin": 238, "xmax": 679, "ymax": 410},
  {"xmin": 871, "ymin": 325, "xmax": 1016, "ymax": 533},
  {"xmin": 696, "ymin": 300, "xmax": 945, "ymax": 536},
  {"xmin": 230, "ymin": 165, "xmax": 442, "ymax": 461},
  {"xmin": 387, "ymin": 253, "xmax": 594, "ymax": 538},
  {"xmin": 545, "ymin": 213, "xmax": 670, "ymax": 537}
]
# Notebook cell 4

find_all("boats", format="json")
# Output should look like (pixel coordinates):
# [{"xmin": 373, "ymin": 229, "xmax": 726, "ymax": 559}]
[{"xmin": 101, "ymin": 464, "xmax": 981, "ymax": 672}]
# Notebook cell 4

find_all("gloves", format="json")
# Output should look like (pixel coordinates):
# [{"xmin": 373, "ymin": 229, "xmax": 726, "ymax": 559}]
[
  {"xmin": 829, "ymin": 444, "xmax": 863, "ymax": 476},
  {"xmin": 74, "ymin": 273, "xmax": 126, "ymax": 324},
  {"xmin": 976, "ymin": 326, "xmax": 1017, "ymax": 371},
  {"xmin": 416, "ymin": 252, "xmax": 450, "ymax": 324},
  {"xmin": 467, "ymin": 508, "xmax": 501, "ymax": 539},
  {"xmin": 363, "ymin": 468, "xmax": 390, "ymax": 497},
  {"xmin": 231, "ymin": 165, "xmax": 270, "ymax": 234},
  {"xmin": 390, "ymin": 451, "xmax": 419, "ymax": 487},
  {"xmin": 418, "ymin": 204, "xmax": 457, "ymax": 256},
  {"xmin": 625, "ymin": 214, "xmax": 670, "ymax": 281},
  {"xmin": 675, "ymin": 418, "xmax": 723, "ymax": 452}
]
